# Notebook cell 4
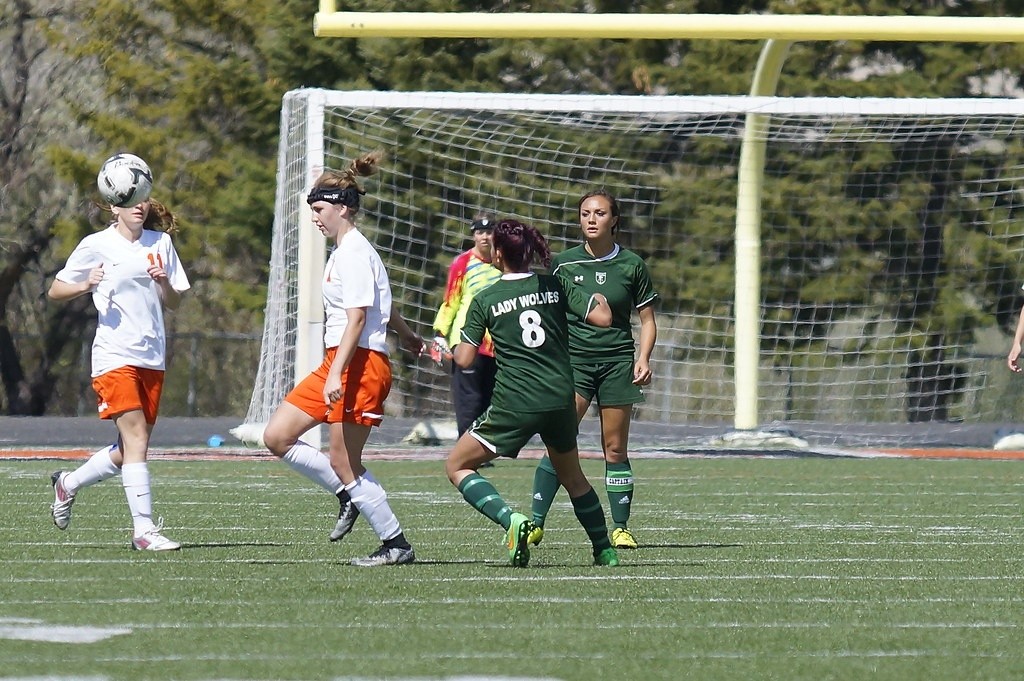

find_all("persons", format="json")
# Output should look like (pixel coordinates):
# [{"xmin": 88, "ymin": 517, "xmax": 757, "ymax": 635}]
[
  {"xmin": 48, "ymin": 196, "xmax": 191, "ymax": 551},
  {"xmin": 263, "ymin": 156, "xmax": 427, "ymax": 567},
  {"xmin": 1007, "ymin": 285, "xmax": 1024, "ymax": 372},
  {"xmin": 429, "ymin": 188, "xmax": 657, "ymax": 568}
]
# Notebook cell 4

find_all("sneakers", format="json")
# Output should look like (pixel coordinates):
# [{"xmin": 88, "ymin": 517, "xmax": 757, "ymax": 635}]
[
  {"xmin": 593, "ymin": 548, "xmax": 620, "ymax": 567},
  {"xmin": 328, "ymin": 499, "xmax": 360, "ymax": 542},
  {"xmin": 352, "ymin": 545, "xmax": 415, "ymax": 567},
  {"xmin": 50, "ymin": 470, "xmax": 77, "ymax": 532},
  {"xmin": 531, "ymin": 523, "xmax": 544, "ymax": 547},
  {"xmin": 610, "ymin": 528, "xmax": 638, "ymax": 550},
  {"xmin": 130, "ymin": 516, "xmax": 181, "ymax": 552},
  {"xmin": 501, "ymin": 512, "xmax": 534, "ymax": 568}
]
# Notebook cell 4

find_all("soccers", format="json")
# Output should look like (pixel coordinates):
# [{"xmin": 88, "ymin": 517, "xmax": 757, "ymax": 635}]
[{"xmin": 95, "ymin": 151, "xmax": 154, "ymax": 209}]
